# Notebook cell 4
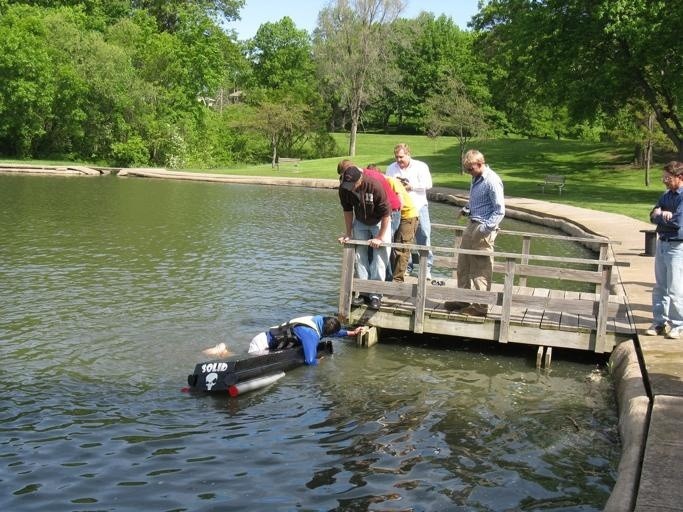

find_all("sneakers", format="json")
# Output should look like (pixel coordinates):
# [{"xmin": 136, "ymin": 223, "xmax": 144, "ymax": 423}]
[
  {"xmin": 667, "ymin": 329, "xmax": 683, "ymax": 339},
  {"xmin": 445, "ymin": 301, "xmax": 487, "ymax": 316},
  {"xmin": 644, "ymin": 324, "xmax": 668, "ymax": 336},
  {"xmin": 405, "ymin": 267, "xmax": 432, "ymax": 280}
]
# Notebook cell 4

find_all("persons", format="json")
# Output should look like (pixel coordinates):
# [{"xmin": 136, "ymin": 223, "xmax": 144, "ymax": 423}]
[
  {"xmin": 202, "ymin": 313, "xmax": 364, "ymax": 365},
  {"xmin": 384, "ymin": 143, "xmax": 433, "ymax": 281},
  {"xmin": 443, "ymin": 147, "xmax": 505, "ymax": 317},
  {"xmin": 335, "ymin": 158, "xmax": 419, "ymax": 312},
  {"xmin": 643, "ymin": 160, "xmax": 683, "ymax": 340}
]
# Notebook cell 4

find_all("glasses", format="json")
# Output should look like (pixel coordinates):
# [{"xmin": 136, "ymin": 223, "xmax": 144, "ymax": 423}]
[
  {"xmin": 664, "ymin": 175, "xmax": 676, "ymax": 182},
  {"xmin": 467, "ymin": 164, "xmax": 477, "ymax": 172}
]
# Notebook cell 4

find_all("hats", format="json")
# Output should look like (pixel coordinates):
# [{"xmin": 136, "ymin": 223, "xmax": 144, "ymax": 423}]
[{"xmin": 340, "ymin": 166, "xmax": 361, "ymax": 192}]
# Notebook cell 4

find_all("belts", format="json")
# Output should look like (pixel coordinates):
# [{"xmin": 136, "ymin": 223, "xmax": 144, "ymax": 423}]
[{"xmin": 659, "ymin": 236, "xmax": 682, "ymax": 242}]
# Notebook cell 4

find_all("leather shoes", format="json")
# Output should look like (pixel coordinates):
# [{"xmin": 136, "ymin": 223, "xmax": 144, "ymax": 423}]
[{"xmin": 352, "ymin": 295, "xmax": 381, "ymax": 310}]
